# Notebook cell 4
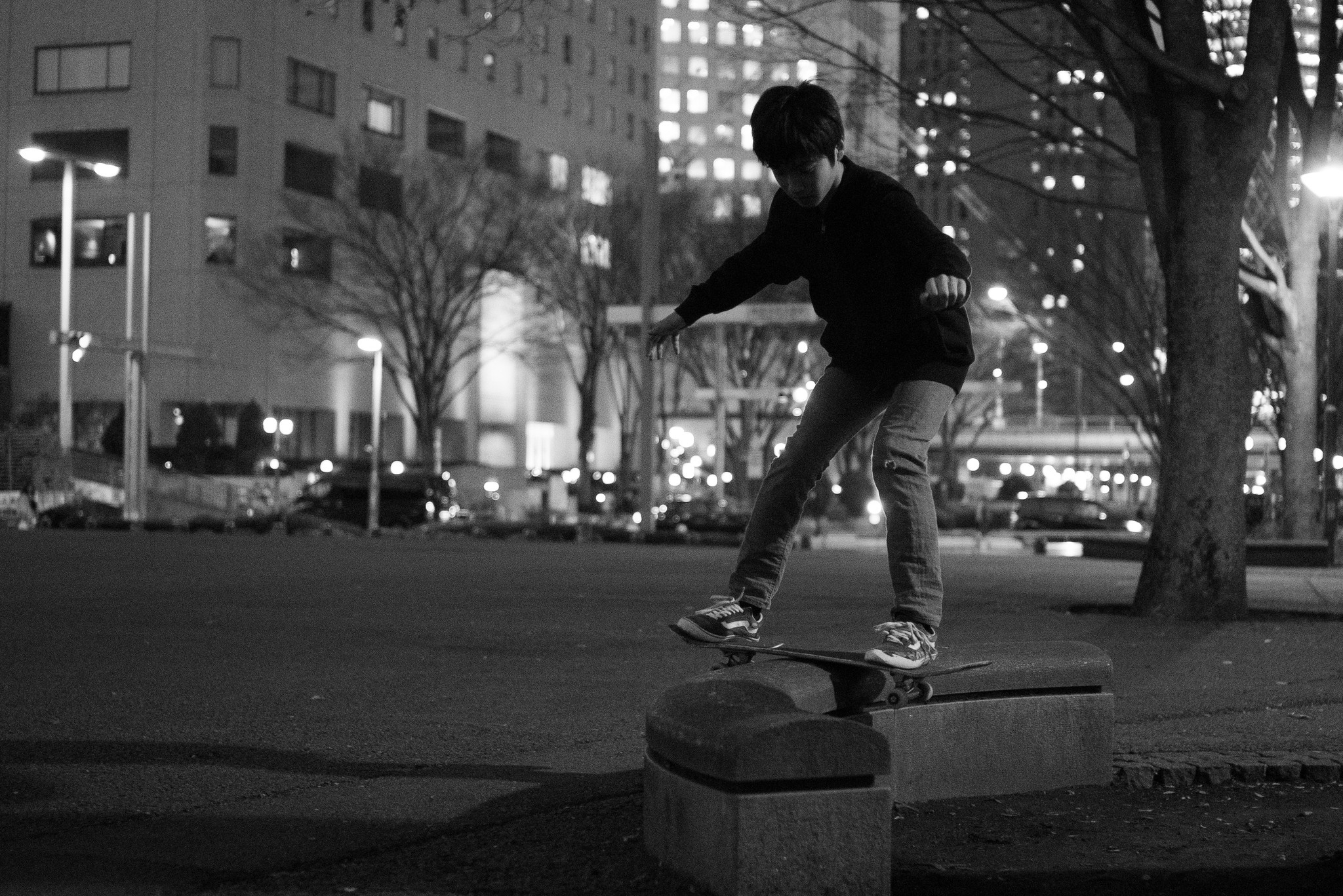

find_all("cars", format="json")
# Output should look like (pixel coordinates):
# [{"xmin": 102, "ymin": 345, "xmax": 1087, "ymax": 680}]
[{"xmin": 1009, "ymin": 495, "xmax": 1139, "ymax": 536}]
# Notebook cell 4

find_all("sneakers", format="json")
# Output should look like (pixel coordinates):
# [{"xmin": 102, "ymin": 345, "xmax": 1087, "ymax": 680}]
[
  {"xmin": 863, "ymin": 621, "xmax": 938, "ymax": 668},
  {"xmin": 676, "ymin": 587, "xmax": 763, "ymax": 642}
]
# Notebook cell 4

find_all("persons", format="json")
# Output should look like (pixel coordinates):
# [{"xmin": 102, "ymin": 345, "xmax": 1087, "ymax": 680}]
[{"xmin": 644, "ymin": 78, "xmax": 977, "ymax": 670}]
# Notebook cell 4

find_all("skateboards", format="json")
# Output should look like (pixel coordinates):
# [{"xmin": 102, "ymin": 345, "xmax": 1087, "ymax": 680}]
[{"xmin": 666, "ymin": 623, "xmax": 996, "ymax": 708}]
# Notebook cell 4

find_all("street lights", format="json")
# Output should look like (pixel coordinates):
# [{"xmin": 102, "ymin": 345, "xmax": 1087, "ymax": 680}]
[
  {"xmin": 14, "ymin": 144, "xmax": 122, "ymax": 449},
  {"xmin": 1296, "ymin": 164, "xmax": 1343, "ymax": 569},
  {"xmin": 357, "ymin": 338, "xmax": 383, "ymax": 536}
]
